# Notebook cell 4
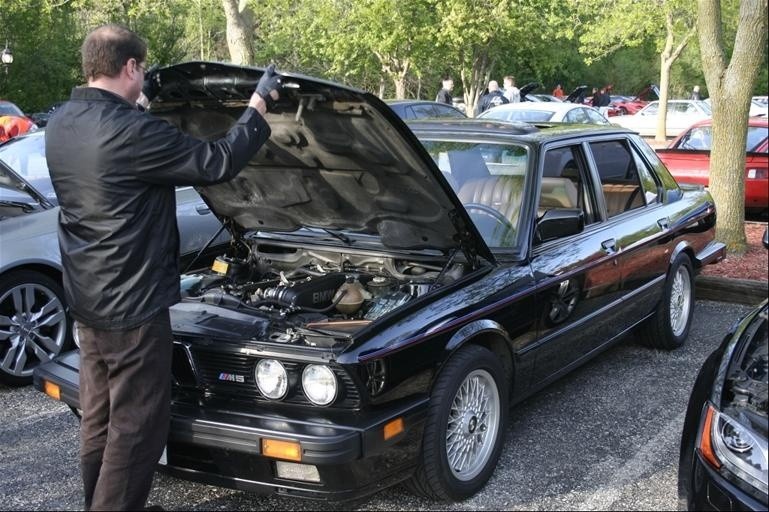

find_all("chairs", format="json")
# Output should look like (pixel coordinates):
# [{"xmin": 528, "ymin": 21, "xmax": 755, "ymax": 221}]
[{"xmin": 458, "ymin": 174, "xmax": 641, "ymax": 247}]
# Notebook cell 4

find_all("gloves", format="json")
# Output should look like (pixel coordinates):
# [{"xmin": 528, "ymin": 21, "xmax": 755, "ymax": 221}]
[
  {"xmin": 255, "ymin": 63, "xmax": 284, "ymax": 113},
  {"xmin": 142, "ymin": 62, "xmax": 163, "ymax": 101}
]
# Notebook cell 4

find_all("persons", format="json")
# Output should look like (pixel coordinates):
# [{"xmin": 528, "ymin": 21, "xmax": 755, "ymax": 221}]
[
  {"xmin": 0, "ymin": 115, "xmax": 38, "ymax": 143},
  {"xmin": 690, "ymin": 85, "xmax": 700, "ymax": 100},
  {"xmin": 552, "ymin": 83, "xmax": 563, "ymax": 97},
  {"xmin": 591, "ymin": 88, "xmax": 610, "ymax": 107},
  {"xmin": 502, "ymin": 75, "xmax": 521, "ymax": 103},
  {"xmin": 475, "ymin": 79, "xmax": 509, "ymax": 114},
  {"xmin": 44, "ymin": 24, "xmax": 284, "ymax": 512},
  {"xmin": 434, "ymin": 77, "xmax": 455, "ymax": 107}
]
